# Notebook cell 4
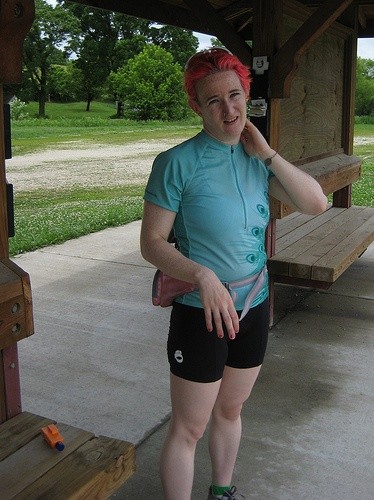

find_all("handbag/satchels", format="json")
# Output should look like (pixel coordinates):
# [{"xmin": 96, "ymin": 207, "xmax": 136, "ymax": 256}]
[{"xmin": 152, "ymin": 267, "xmax": 197, "ymax": 308}]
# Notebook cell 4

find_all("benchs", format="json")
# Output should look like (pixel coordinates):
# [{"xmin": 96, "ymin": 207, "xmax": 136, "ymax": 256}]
[
  {"xmin": 0, "ymin": 405, "xmax": 136, "ymax": 500},
  {"xmin": 254, "ymin": 200, "xmax": 374, "ymax": 289}
]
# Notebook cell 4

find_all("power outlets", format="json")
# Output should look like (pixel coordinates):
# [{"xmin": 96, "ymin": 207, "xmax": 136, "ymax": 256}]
[
  {"xmin": 251, "ymin": 56, "xmax": 268, "ymax": 75},
  {"xmin": 248, "ymin": 100, "xmax": 267, "ymax": 119}
]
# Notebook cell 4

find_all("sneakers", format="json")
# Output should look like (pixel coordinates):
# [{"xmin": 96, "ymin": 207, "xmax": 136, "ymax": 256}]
[{"xmin": 208, "ymin": 485, "xmax": 246, "ymax": 500}]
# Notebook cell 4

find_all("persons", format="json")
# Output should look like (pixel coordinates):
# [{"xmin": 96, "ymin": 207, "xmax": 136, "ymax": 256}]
[{"xmin": 140, "ymin": 49, "xmax": 330, "ymax": 500}]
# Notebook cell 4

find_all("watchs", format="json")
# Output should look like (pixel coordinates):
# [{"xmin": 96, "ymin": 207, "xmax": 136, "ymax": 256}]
[{"xmin": 261, "ymin": 151, "xmax": 280, "ymax": 168}]
